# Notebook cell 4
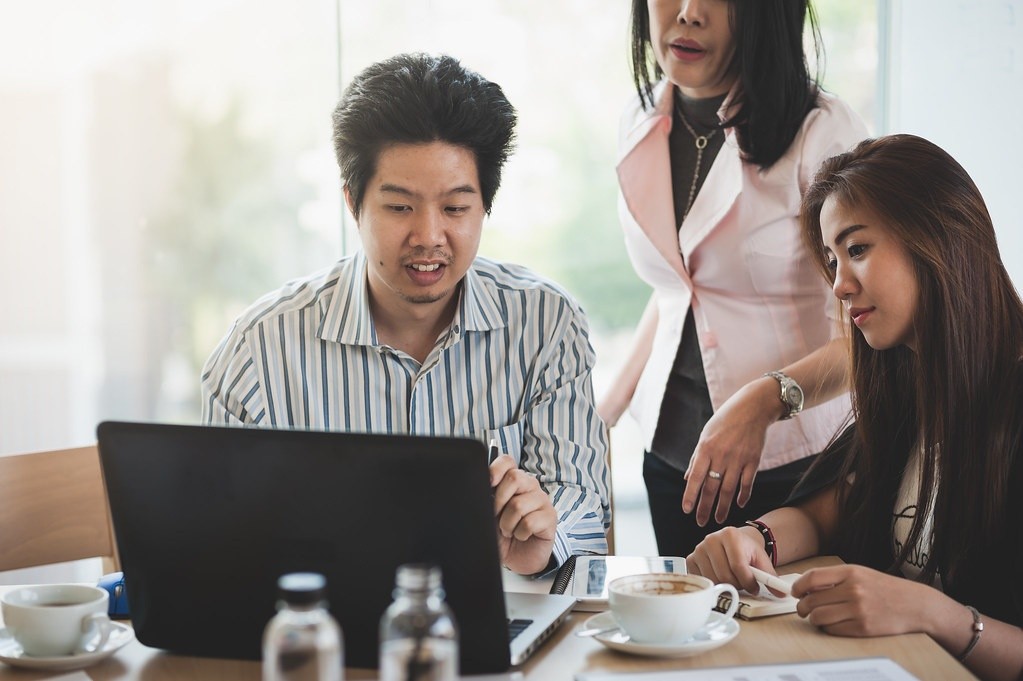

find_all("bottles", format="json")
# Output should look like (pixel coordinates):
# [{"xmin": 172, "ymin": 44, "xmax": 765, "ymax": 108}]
[
  {"xmin": 379, "ymin": 564, "xmax": 461, "ymax": 681},
  {"xmin": 262, "ymin": 572, "xmax": 345, "ymax": 681}
]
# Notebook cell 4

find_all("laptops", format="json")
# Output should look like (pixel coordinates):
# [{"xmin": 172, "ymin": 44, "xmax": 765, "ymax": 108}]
[{"xmin": 96, "ymin": 419, "xmax": 578, "ymax": 679}]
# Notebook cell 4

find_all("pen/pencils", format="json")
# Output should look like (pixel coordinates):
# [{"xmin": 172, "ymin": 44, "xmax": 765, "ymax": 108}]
[
  {"xmin": 489, "ymin": 438, "xmax": 499, "ymax": 465},
  {"xmin": 749, "ymin": 565, "xmax": 794, "ymax": 593}
]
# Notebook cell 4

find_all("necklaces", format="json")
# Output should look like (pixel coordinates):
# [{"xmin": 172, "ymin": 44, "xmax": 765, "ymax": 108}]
[{"xmin": 673, "ymin": 100, "xmax": 720, "ymax": 226}]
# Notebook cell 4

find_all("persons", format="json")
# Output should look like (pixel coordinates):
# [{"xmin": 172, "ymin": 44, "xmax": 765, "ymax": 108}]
[
  {"xmin": 198, "ymin": 50, "xmax": 611, "ymax": 582},
  {"xmin": 685, "ymin": 133, "xmax": 1023, "ymax": 680},
  {"xmin": 594, "ymin": 0, "xmax": 873, "ymax": 555}
]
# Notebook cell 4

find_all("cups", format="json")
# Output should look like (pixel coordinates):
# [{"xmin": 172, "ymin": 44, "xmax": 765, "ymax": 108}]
[
  {"xmin": 1, "ymin": 583, "xmax": 112, "ymax": 653},
  {"xmin": 609, "ymin": 573, "xmax": 739, "ymax": 641}
]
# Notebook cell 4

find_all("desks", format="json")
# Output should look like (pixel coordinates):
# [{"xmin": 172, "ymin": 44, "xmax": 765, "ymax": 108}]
[{"xmin": 0, "ymin": 550, "xmax": 980, "ymax": 681}]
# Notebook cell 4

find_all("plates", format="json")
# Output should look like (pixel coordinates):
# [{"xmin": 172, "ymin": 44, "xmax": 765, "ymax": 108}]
[
  {"xmin": 0, "ymin": 615, "xmax": 135, "ymax": 668},
  {"xmin": 585, "ymin": 611, "xmax": 740, "ymax": 658}
]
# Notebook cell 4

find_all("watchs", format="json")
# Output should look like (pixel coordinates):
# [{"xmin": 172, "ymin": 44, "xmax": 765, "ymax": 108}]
[{"xmin": 762, "ymin": 371, "xmax": 804, "ymax": 422}]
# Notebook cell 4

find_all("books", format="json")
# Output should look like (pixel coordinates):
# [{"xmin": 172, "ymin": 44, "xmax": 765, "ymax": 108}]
[
  {"xmin": 574, "ymin": 657, "xmax": 920, "ymax": 681},
  {"xmin": 713, "ymin": 572, "xmax": 802, "ymax": 622},
  {"xmin": 500, "ymin": 555, "xmax": 688, "ymax": 613}
]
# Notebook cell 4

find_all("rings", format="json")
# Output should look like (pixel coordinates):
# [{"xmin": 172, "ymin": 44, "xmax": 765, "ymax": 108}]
[{"xmin": 706, "ymin": 469, "xmax": 721, "ymax": 480}]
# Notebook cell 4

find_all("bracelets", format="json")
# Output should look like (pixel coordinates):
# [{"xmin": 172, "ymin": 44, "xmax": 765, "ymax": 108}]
[
  {"xmin": 957, "ymin": 604, "xmax": 983, "ymax": 662},
  {"xmin": 743, "ymin": 519, "xmax": 779, "ymax": 570}
]
